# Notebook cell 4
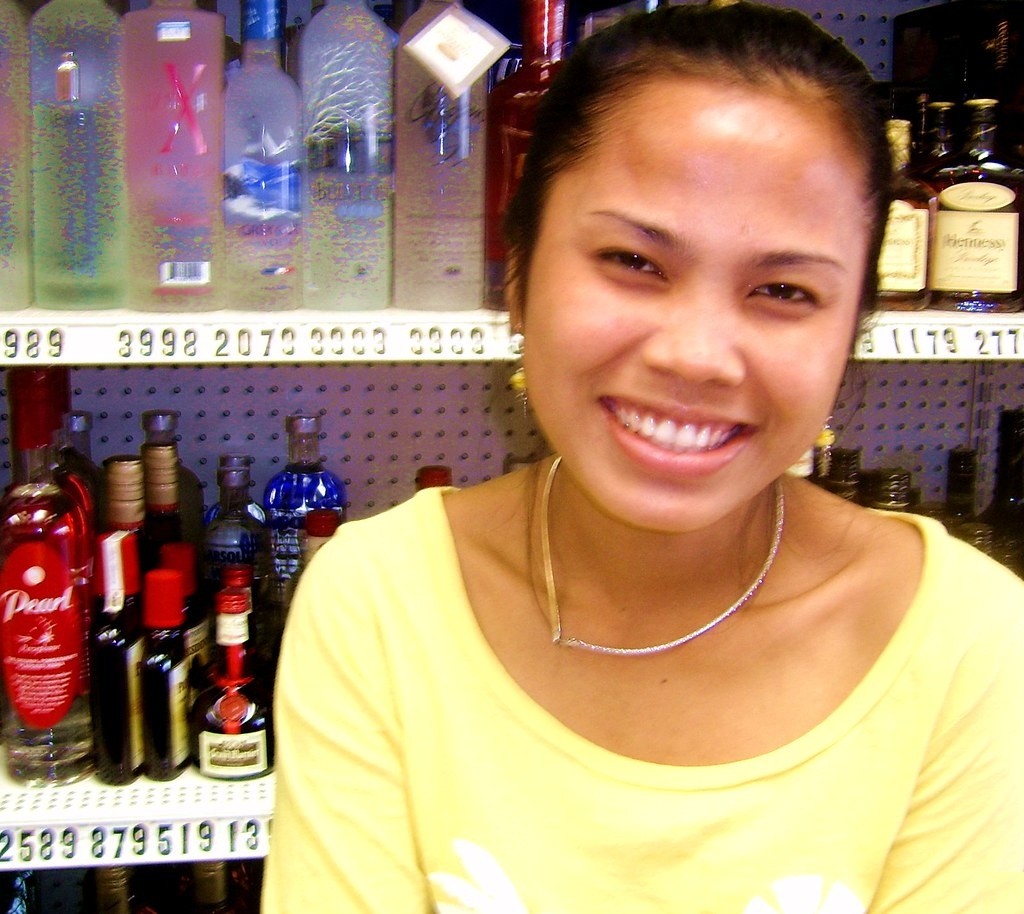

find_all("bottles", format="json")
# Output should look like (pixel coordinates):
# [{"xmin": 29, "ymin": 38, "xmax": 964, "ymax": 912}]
[
  {"xmin": 7, "ymin": 368, "xmax": 348, "ymax": 804},
  {"xmin": 5, "ymin": 0, "xmax": 596, "ymax": 312},
  {"xmin": 866, "ymin": 74, "xmax": 1024, "ymax": 315},
  {"xmin": 814, "ymin": 404, "xmax": 1022, "ymax": 597}
]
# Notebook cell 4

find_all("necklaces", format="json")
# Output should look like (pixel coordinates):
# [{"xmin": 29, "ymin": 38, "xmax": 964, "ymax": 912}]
[{"xmin": 523, "ymin": 457, "xmax": 784, "ymax": 657}]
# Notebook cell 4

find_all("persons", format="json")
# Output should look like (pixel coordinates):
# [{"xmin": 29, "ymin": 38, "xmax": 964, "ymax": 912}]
[{"xmin": 260, "ymin": 1, "xmax": 1024, "ymax": 914}]
